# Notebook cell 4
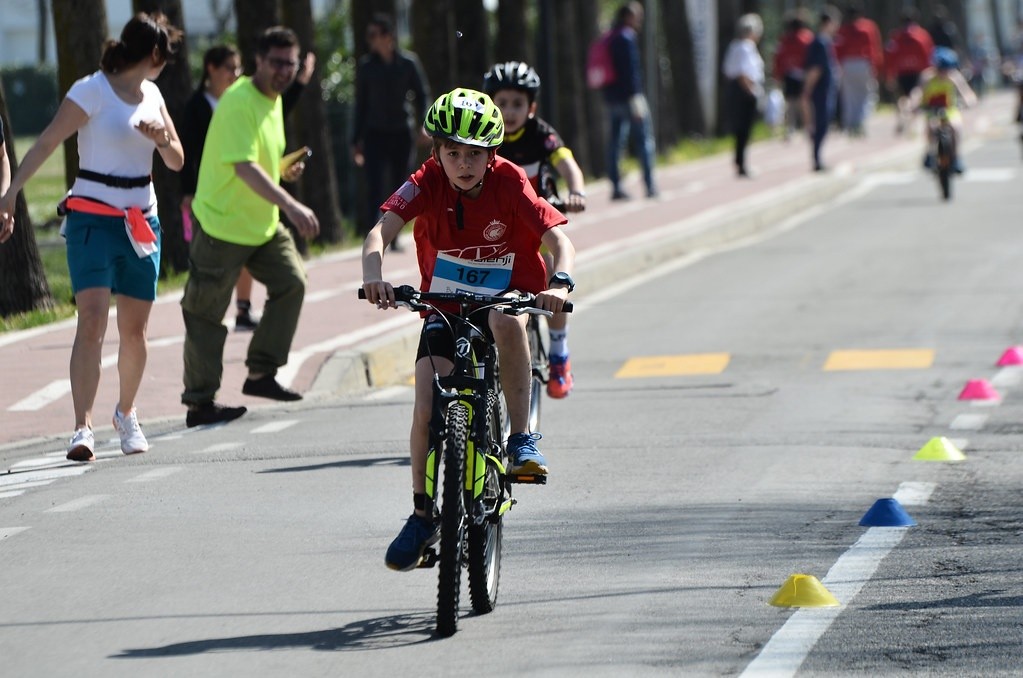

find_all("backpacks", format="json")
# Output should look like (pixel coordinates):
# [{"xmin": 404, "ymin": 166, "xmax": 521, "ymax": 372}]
[{"xmin": 585, "ymin": 27, "xmax": 621, "ymax": 88}]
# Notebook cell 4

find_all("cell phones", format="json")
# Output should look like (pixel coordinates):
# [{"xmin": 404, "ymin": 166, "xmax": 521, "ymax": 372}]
[{"xmin": 292, "ymin": 150, "xmax": 312, "ymax": 166}]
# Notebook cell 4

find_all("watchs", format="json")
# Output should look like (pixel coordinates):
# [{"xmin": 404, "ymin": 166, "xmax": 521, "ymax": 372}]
[{"xmin": 548, "ymin": 271, "xmax": 574, "ymax": 293}]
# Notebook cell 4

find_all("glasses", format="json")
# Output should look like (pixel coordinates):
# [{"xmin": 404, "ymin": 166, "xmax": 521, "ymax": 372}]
[
  {"xmin": 262, "ymin": 53, "xmax": 298, "ymax": 69},
  {"xmin": 223, "ymin": 62, "xmax": 243, "ymax": 76},
  {"xmin": 366, "ymin": 31, "xmax": 383, "ymax": 39}
]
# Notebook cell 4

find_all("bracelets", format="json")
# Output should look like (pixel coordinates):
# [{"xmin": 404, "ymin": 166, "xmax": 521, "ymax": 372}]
[
  {"xmin": 159, "ymin": 137, "xmax": 170, "ymax": 147},
  {"xmin": 569, "ymin": 190, "xmax": 587, "ymax": 197}
]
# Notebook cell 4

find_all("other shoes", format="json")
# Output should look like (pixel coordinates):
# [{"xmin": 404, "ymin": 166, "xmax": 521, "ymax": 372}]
[
  {"xmin": 925, "ymin": 155, "xmax": 933, "ymax": 169},
  {"xmin": 955, "ymin": 164, "xmax": 961, "ymax": 176},
  {"xmin": 234, "ymin": 314, "xmax": 260, "ymax": 332}
]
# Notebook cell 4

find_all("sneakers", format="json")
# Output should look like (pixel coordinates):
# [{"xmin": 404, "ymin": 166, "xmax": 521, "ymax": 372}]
[
  {"xmin": 546, "ymin": 352, "xmax": 573, "ymax": 398},
  {"xmin": 66, "ymin": 426, "xmax": 93, "ymax": 459},
  {"xmin": 186, "ymin": 401, "xmax": 246, "ymax": 428},
  {"xmin": 385, "ymin": 507, "xmax": 441, "ymax": 572},
  {"xmin": 506, "ymin": 433, "xmax": 549, "ymax": 475},
  {"xmin": 111, "ymin": 402, "xmax": 148, "ymax": 454},
  {"xmin": 242, "ymin": 373, "xmax": 303, "ymax": 401}
]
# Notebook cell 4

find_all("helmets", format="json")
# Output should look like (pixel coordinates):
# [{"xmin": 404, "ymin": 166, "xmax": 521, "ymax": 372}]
[
  {"xmin": 484, "ymin": 61, "xmax": 541, "ymax": 106},
  {"xmin": 424, "ymin": 87, "xmax": 505, "ymax": 147},
  {"xmin": 932, "ymin": 48, "xmax": 959, "ymax": 69}
]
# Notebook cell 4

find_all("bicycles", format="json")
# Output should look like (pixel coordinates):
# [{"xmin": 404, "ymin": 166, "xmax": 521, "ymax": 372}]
[
  {"xmin": 937, "ymin": 124, "xmax": 953, "ymax": 198},
  {"xmin": 495, "ymin": 202, "xmax": 585, "ymax": 455},
  {"xmin": 358, "ymin": 285, "xmax": 573, "ymax": 635}
]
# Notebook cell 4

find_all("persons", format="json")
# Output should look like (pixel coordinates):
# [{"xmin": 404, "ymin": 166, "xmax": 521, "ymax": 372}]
[
  {"xmin": 481, "ymin": 60, "xmax": 587, "ymax": 397},
  {"xmin": 0, "ymin": 11, "xmax": 185, "ymax": 463},
  {"xmin": 719, "ymin": 7, "xmax": 934, "ymax": 178},
  {"xmin": 360, "ymin": 89, "xmax": 575, "ymax": 572},
  {"xmin": 349, "ymin": 12, "xmax": 429, "ymax": 250},
  {"xmin": 908, "ymin": 46, "xmax": 976, "ymax": 172},
  {"xmin": 174, "ymin": 42, "xmax": 315, "ymax": 331},
  {"xmin": 598, "ymin": 0, "xmax": 659, "ymax": 201},
  {"xmin": 179, "ymin": 26, "xmax": 322, "ymax": 427}
]
